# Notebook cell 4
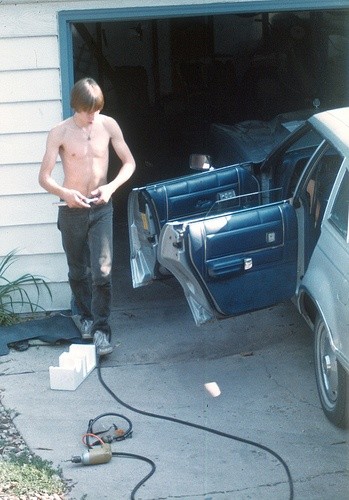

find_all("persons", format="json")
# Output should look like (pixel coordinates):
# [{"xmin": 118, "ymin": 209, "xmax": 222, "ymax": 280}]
[
  {"xmin": 307, "ymin": 180, "xmax": 324, "ymax": 227},
  {"xmin": 38, "ymin": 78, "xmax": 136, "ymax": 357}
]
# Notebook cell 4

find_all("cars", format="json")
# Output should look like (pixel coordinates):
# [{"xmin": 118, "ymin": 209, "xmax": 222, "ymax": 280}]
[{"xmin": 126, "ymin": 96, "xmax": 349, "ymax": 427}]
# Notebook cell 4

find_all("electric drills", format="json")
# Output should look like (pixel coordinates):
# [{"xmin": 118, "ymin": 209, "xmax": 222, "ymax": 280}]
[{"xmin": 60, "ymin": 443, "xmax": 112, "ymax": 466}]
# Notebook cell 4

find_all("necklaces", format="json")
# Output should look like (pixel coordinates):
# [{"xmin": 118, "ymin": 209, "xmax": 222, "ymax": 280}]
[{"xmin": 71, "ymin": 115, "xmax": 93, "ymax": 140}]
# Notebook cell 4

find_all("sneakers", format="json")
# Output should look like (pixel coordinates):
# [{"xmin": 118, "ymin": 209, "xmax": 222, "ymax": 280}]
[
  {"xmin": 93, "ymin": 330, "xmax": 114, "ymax": 355},
  {"xmin": 80, "ymin": 320, "xmax": 93, "ymax": 339}
]
